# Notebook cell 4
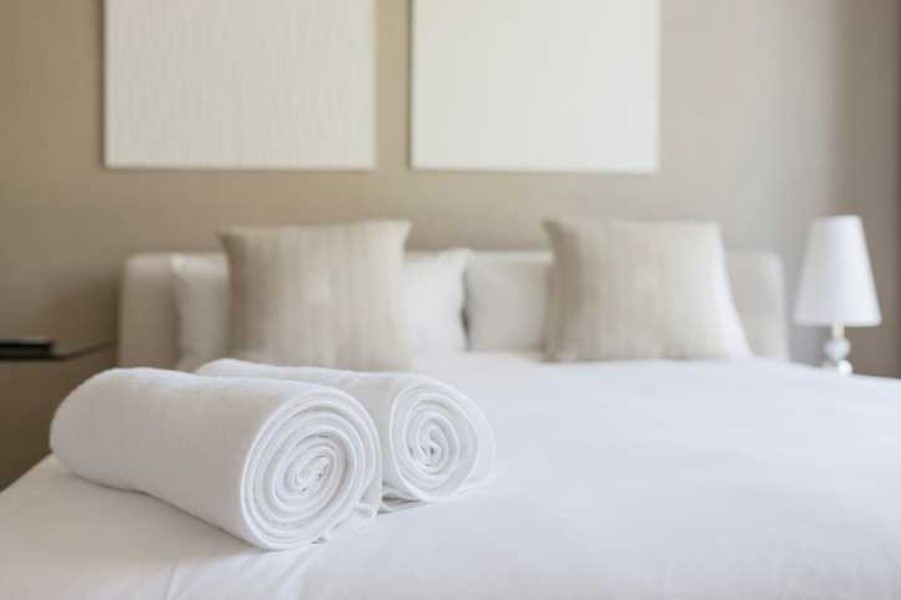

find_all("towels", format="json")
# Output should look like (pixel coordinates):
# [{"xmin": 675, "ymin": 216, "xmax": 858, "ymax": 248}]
[
  {"xmin": 49, "ymin": 366, "xmax": 384, "ymax": 553},
  {"xmin": 193, "ymin": 357, "xmax": 496, "ymax": 505}
]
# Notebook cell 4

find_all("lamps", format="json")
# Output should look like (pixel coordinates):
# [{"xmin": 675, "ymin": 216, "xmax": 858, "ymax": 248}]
[{"xmin": 791, "ymin": 213, "xmax": 883, "ymax": 374}]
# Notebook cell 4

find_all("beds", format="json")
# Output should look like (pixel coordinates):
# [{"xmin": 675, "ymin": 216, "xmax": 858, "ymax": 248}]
[{"xmin": 0, "ymin": 248, "xmax": 901, "ymax": 600}]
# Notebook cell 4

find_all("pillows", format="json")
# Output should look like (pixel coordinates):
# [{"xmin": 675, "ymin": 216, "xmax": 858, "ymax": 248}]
[
  {"xmin": 220, "ymin": 217, "xmax": 412, "ymax": 377},
  {"xmin": 169, "ymin": 244, "xmax": 470, "ymax": 375},
  {"xmin": 548, "ymin": 215, "xmax": 752, "ymax": 364}
]
